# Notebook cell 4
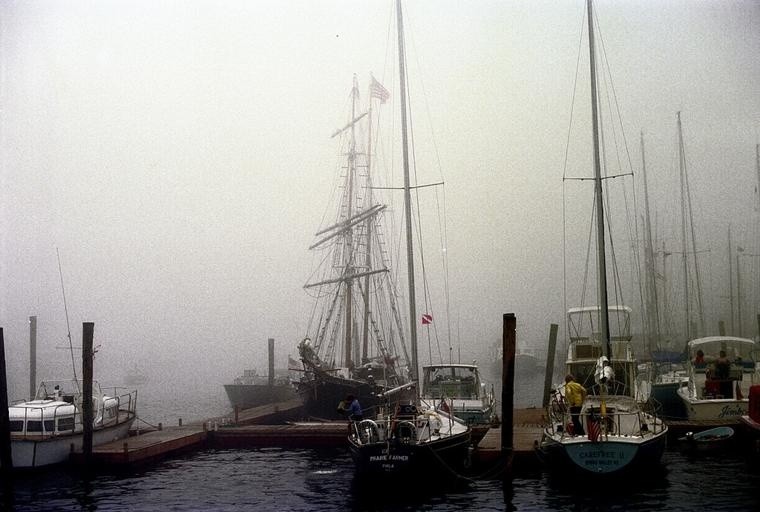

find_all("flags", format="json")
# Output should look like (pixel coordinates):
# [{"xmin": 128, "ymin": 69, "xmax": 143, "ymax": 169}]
[{"xmin": 421, "ymin": 314, "xmax": 433, "ymax": 324}]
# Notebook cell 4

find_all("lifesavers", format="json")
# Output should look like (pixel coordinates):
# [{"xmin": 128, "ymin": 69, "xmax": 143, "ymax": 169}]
[
  {"xmin": 396, "ymin": 421, "xmax": 417, "ymax": 444},
  {"xmin": 359, "ymin": 420, "xmax": 379, "ymax": 444},
  {"xmin": 36, "ymin": 387, "xmax": 47, "ymax": 399}
]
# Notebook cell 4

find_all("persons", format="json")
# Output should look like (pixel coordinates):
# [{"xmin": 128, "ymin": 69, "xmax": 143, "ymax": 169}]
[
  {"xmin": 340, "ymin": 392, "xmax": 365, "ymax": 421},
  {"xmin": 716, "ymin": 348, "xmax": 731, "ymax": 378},
  {"xmin": 694, "ymin": 349, "xmax": 706, "ymax": 369},
  {"xmin": 564, "ymin": 375, "xmax": 588, "ymax": 434}
]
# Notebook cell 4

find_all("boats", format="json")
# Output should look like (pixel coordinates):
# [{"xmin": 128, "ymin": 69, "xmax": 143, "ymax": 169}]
[{"xmin": 9, "ymin": 378, "xmax": 138, "ymax": 469}]
